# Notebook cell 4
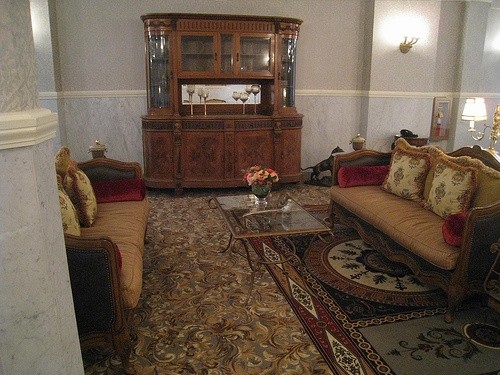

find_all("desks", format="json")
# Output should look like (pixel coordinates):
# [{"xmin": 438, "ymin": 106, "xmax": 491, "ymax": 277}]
[{"xmin": 208, "ymin": 191, "xmax": 332, "ymax": 308}]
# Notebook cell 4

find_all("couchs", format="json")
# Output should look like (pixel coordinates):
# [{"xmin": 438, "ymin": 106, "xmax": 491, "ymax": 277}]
[
  {"xmin": 329, "ymin": 134, "xmax": 500, "ymax": 323},
  {"xmin": 55, "ymin": 140, "xmax": 150, "ymax": 375}
]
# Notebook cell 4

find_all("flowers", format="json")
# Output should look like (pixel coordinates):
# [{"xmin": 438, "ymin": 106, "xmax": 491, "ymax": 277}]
[{"xmin": 241, "ymin": 166, "xmax": 279, "ymax": 185}]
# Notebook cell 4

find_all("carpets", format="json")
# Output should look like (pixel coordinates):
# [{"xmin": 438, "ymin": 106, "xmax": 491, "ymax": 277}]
[{"xmin": 231, "ymin": 205, "xmax": 500, "ymax": 375}]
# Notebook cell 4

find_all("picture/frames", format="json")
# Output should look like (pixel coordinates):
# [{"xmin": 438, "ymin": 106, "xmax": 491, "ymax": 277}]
[{"xmin": 430, "ymin": 97, "xmax": 453, "ymax": 141}]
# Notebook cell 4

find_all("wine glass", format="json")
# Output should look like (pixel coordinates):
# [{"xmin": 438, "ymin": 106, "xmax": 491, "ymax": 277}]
[
  {"xmin": 240, "ymin": 84, "xmax": 261, "ymax": 115},
  {"xmin": 232, "ymin": 85, "xmax": 252, "ymax": 103},
  {"xmin": 187, "ymin": 84, "xmax": 209, "ymax": 115},
  {"xmin": 197, "ymin": 88, "xmax": 202, "ymax": 104}
]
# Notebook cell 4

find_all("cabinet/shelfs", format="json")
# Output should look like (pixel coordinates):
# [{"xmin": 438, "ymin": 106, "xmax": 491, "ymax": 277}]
[{"xmin": 140, "ymin": 13, "xmax": 304, "ymax": 198}]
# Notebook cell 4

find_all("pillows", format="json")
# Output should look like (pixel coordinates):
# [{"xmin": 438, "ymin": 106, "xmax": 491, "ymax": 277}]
[
  {"xmin": 424, "ymin": 157, "xmax": 478, "ymax": 221},
  {"xmin": 57, "ymin": 160, "xmax": 146, "ymax": 273},
  {"xmin": 381, "ymin": 147, "xmax": 431, "ymax": 202},
  {"xmin": 338, "ymin": 165, "xmax": 390, "ymax": 188},
  {"xmin": 442, "ymin": 213, "xmax": 468, "ymax": 245}
]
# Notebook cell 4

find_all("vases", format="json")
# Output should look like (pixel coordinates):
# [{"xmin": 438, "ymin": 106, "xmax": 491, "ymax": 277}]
[{"xmin": 252, "ymin": 185, "xmax": 272, "ymax": 208}]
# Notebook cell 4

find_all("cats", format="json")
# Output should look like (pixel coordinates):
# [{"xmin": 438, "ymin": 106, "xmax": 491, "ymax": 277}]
[{"xmin": 301, "ymin": 146, "xmax": 344, "ymax": 182}]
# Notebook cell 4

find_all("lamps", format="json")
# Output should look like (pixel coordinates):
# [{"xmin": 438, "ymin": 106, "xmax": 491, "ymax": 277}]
[{"xmin": 461, "ymin": 97, "xmax": 500, "ymax": 150}]
